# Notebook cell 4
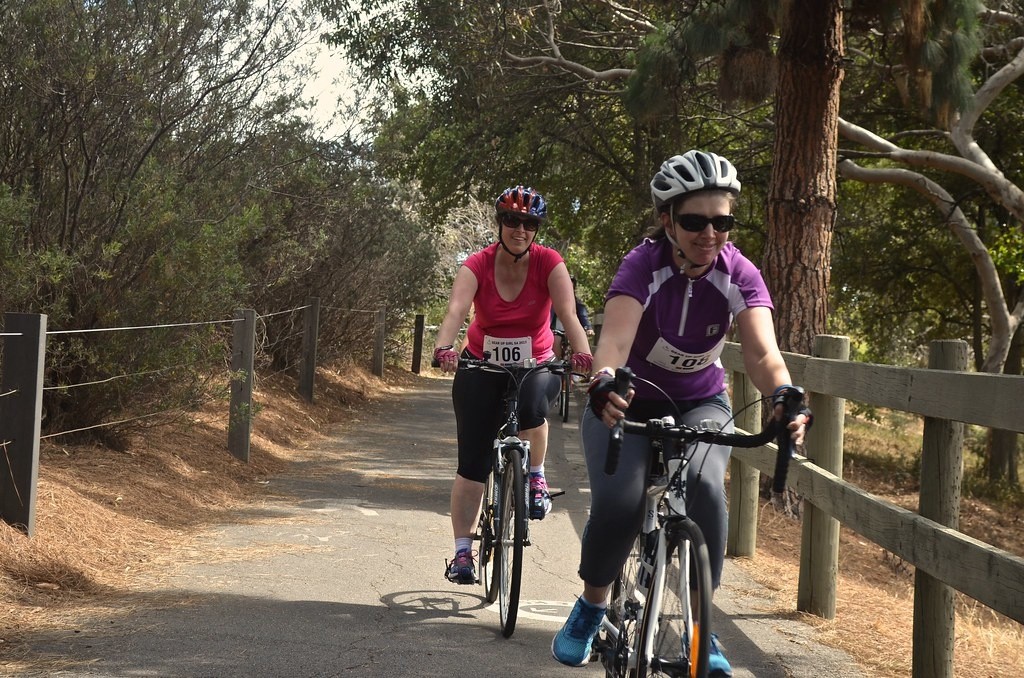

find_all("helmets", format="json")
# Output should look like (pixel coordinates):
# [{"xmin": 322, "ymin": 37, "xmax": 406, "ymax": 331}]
[
  {"xmin": 496, "ymin": 185, "xmax": 548, "ymax": 220},
  {"xmin": 650, "ymin": 148, "xmax": 742, "ymax": 212},
  {"xmin": 569, "ymin": 275, "xmax": 577, "ymax": 287}
]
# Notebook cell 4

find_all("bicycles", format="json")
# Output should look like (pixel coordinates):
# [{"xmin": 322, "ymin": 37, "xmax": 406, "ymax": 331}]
[
  {"xmin": 428, "ymin": 354, "xmax": 588, "ymax": 638},
  {"xmin": 551, "ymin": 328, "xmax": 588, "ymax": 422},
  {"xmin": 595, "ymin": 367, "xmax": 807, "ymax": 677}
]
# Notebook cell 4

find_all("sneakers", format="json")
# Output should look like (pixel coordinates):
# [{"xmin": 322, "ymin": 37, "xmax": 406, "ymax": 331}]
[
  {"xmin": 682, "ymin": 630, "xmax": 732, "ymax": 678},
  {"xmin": 450, "ymin": 548, "xmax": 478, "ymax": 585},
  {"xmin": 551, "ymin": 593, "xmax": 607, "ymax": 667},
  {"xmin": 529, "ymin": 471, "xmax": 552, "ymax": 515}
]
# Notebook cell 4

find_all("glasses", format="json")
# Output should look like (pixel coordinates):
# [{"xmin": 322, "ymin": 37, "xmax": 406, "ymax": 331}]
[
  {"xmin": 502, "ymin": 215, "xmax": 540, "ymax": 231},
  {"xmin": 666, "ymin": 209, "xmax": 734, "ymax": 233}
]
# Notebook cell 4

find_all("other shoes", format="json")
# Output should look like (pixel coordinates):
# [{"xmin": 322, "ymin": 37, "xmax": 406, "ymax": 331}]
[{"xmin": 571, "ymin": 373, "xmax": 580, "ymax": 382}]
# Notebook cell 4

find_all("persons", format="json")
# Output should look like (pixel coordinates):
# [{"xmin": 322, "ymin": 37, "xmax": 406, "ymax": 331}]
[
  {"xmin": 549, "ymin": 274, "xmax": 596, "ymax": 360},
  {"xmin": 551, "ymin": 150, "xmax": 814, "ymax": 678},
  {"xmin": 433, "ymin": 185, "xmax": 593, "ymax": 584}
]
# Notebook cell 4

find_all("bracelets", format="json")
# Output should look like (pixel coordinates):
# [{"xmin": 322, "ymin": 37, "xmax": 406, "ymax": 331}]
[{"xmin": 774, "ymin": 384, "xmax": 793, "ymax": 393}]
